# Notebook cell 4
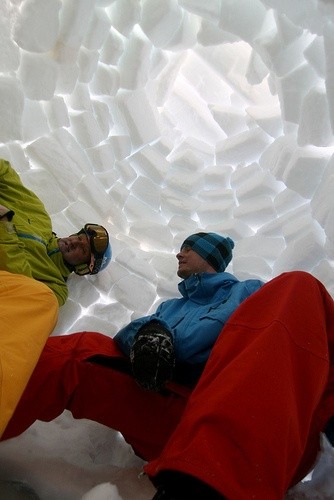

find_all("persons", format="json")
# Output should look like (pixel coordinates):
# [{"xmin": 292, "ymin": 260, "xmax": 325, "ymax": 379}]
[
  {"xmin": 0, "ymin": 160, "xmax": 112, "ymax": 436},
  {"xmin": 0, "ymin": 231, "xmax": 334, "ymax": 500}
]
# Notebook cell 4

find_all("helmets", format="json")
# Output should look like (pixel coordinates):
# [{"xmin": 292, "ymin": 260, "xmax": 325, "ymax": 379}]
[{"xmin": 78, "ymin": 227, "xmax": 112, "ymax": 272}]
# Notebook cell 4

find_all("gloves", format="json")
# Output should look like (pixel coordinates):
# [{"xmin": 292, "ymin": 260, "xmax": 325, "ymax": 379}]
[{"xmin": 130, "ymin": 325, "xmax": 175, "ymax": 392}]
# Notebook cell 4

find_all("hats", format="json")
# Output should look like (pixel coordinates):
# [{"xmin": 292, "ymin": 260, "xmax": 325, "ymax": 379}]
[{"xmin": 180, "ymin": 232, "xmax": 234, "ymax": 273}]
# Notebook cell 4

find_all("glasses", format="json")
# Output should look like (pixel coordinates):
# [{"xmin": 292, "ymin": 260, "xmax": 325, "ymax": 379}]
[{"xmin": 84, "ymin": 223, "xmax": 109, "ymax": 274}]
